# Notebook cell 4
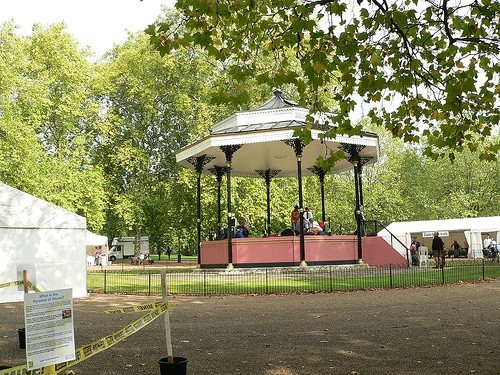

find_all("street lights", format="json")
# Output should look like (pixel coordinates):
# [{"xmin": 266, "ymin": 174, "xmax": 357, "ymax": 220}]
[{"xmin": 176, "ymin": 227, "xmax": 183, "ymax": 263}]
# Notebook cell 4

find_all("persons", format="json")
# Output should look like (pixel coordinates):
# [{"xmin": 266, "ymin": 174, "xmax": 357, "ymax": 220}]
[
  {"xmin": 450, "ymin": 235, "xmax": 500, "ymax": 257},
  {"xmin": 137, "ymin": 214, "xmax": 249, "ymax": 264},
  {"xmin": 290, "ymin": 205, "xmax": 324, "ymax": 234},
  {"xmin": 412, "ymin": 236, "xmax": 420, "ymax": 268},
  {"xmin": 410, "ymin": 244, "xmax": 417, "ymax": 266},
  {"xmin": 441, "ymin": 242, "xmax": 447, "ymax": 268},
  {"xmin": 431, "ymin": 231, "xmax": 443, "ymax": 269}
]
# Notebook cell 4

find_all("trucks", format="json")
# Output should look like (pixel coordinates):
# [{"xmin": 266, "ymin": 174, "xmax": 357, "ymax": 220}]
[{"xmin": 108, "ymin": 235, "xmax": 150, "ymax": 262}]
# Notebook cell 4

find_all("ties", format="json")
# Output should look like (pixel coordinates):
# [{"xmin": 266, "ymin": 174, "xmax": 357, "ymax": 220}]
[{"xmin": 307, "ymin": 212, "xmax": 308, "ymax": 219}]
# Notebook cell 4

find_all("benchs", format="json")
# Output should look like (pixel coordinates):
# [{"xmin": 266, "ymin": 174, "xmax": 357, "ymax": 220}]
[{"xmin": 131, "ymin": 257, "xmax": 154, "ymax": 266}]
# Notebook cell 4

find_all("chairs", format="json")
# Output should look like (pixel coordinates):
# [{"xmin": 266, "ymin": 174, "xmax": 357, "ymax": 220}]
[{"xmin": 418, "ymin": 246, "xmax": 429, "ymax": 269}]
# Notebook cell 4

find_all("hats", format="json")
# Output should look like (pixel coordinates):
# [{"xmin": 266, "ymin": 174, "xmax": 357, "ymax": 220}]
[
  {"xmin": 305, "ymin": 207, "xmax": 309, "ymax": 210},
  {"xmin": 294, "ymin": 205, "xmax": 299, "ymax": 209}
]
